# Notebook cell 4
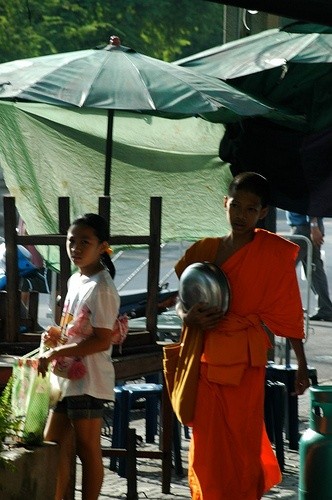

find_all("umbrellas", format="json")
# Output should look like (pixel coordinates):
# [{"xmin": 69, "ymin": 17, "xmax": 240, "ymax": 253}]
[{"xmin": 0, "ymin": 29, "xmax": 332, "ymax": 123}]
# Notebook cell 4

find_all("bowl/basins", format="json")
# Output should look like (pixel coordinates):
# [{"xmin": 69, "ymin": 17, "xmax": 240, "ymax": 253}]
[{"xmin": 179, "ymin": 261, "xmax": 231, "ymax": 318}]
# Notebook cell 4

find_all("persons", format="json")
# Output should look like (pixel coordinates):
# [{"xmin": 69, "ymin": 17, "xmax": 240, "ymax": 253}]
[
  {"xmin": 0, "ymin": 237, "xmax": 49, "ymax": 335},
  {"xmin": 281, "ymin": 208, "xmax": 332, "ymax": 321},
  {"xmin": 41, "ymin": 213, "xmax": 121, "ymax": 500},
  {"xmin": 176, "ymin": 172, "xmax": 310, "ymax": 500}
]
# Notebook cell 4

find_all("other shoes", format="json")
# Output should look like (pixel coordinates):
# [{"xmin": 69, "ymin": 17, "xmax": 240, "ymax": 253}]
[{"xmin": 309, "ymin": 312, "xmax": 332, "ymax": 322}]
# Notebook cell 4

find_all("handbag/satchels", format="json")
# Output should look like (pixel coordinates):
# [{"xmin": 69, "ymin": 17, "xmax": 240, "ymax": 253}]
[
  {"xmin": 162, "ymin": 324, "xmax": 205, "ymax": 425},
  {"xmin": 0, "ymin": 343, "xmax": 51, "ymax": 448}
]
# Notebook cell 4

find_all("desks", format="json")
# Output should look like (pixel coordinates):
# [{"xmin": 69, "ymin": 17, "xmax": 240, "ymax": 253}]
[{"xmin": 0, "ymin": 196, "xmax": 179, "ymax": 494}]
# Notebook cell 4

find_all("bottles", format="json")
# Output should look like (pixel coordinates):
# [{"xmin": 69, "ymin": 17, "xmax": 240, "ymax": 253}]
[{"xmin": 42, "ymin": 304, "xmax": 131, "ymax": 380}]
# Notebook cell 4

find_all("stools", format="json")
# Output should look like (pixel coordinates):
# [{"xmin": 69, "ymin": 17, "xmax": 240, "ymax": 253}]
[
  {"xmin": 109, "ymin": 383, "xmax": 184, "ymax": 482},
  {"xmin": 264, "ymin": 362, "xmax": 321, "ymax": 472}
]
